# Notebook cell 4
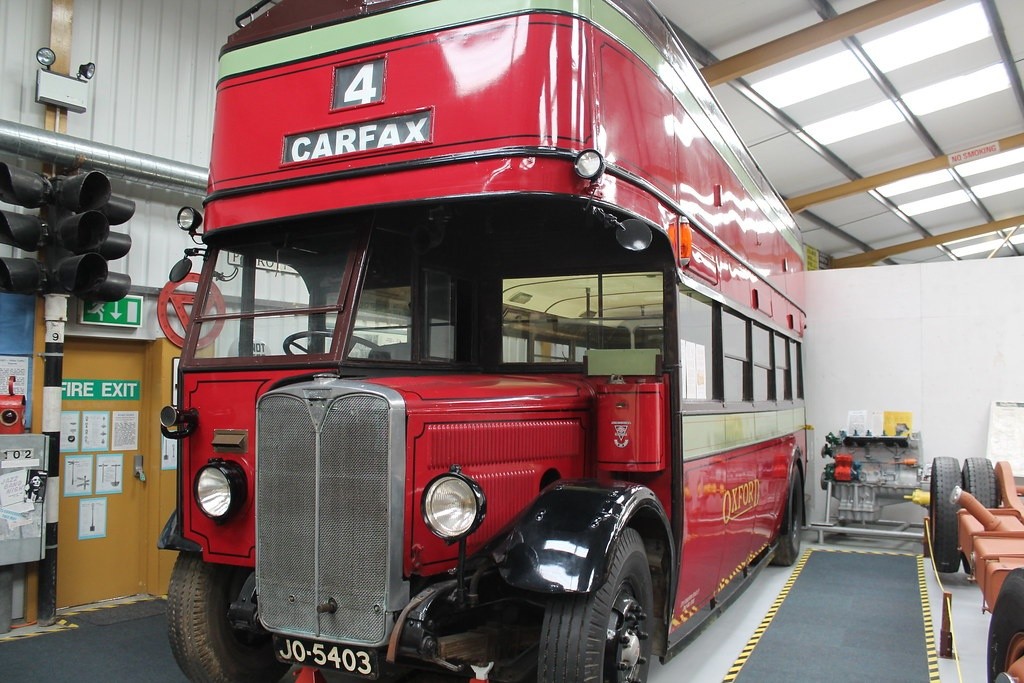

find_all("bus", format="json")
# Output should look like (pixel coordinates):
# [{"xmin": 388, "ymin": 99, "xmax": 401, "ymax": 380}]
[{"xmin": 154, "ymin": 0, "xmax": 810, "ymax": 683}]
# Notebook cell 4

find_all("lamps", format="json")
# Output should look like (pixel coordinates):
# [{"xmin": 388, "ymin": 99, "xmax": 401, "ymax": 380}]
[
  {"xmin": 77, "ymin": 62, "xmax": 95, "ymax": 80},
  {"xmin": 36, "ymin": 47, "xmax": 57, "ymax": 71}
]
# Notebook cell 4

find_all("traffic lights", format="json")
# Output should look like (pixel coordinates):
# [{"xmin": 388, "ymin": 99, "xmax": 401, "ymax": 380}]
[
  {"xmin": 0, "ymin": 162, "xmax": 46, "ymax": 293},
  {"xmin": 48, "ymin": 170, "xmax": 112, "ymax": 291},
  {"xmin": 95, "ymin": 201, "xmax": 136, "ymax": 303}
]
